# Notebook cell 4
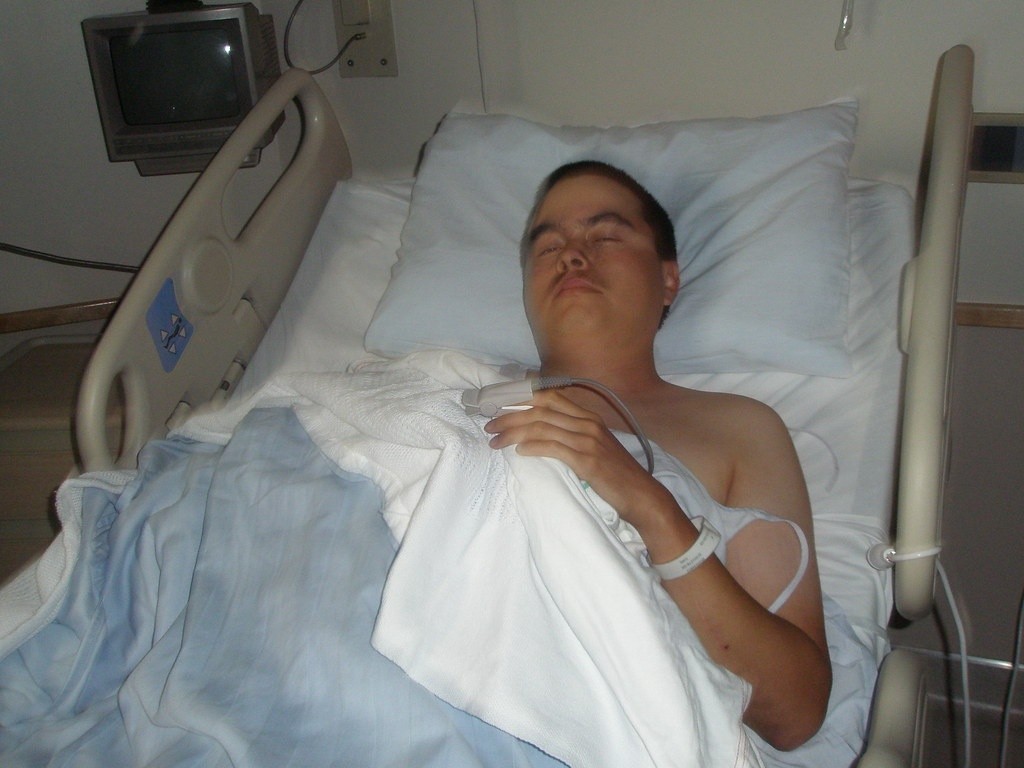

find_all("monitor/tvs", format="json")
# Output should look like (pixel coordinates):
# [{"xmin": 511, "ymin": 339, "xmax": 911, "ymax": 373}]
[{"xmin": 80, "ymin": 3, "xmax": 286, "ymax": 177}]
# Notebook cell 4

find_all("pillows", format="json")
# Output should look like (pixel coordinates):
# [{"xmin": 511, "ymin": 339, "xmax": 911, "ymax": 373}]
[{"xmin": 362, "ymin": 95, "xmax": 860, "ymax": 380}]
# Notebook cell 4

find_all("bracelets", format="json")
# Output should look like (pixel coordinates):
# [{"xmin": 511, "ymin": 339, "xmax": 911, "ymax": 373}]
[{"xmin": 646, "ymin": 515, "xmax": 721, "ymax": 581}]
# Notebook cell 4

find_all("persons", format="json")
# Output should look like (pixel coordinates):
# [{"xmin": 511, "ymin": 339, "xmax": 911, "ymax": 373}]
[{"xmin": 483, "ymin": 161, "xmax": 832, "ymax": 752}]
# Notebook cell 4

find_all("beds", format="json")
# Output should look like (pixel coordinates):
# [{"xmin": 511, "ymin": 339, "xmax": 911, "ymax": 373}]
[{"xmin": 0, "ymin": 44, "xmax": 974, "ymax": 768}]
